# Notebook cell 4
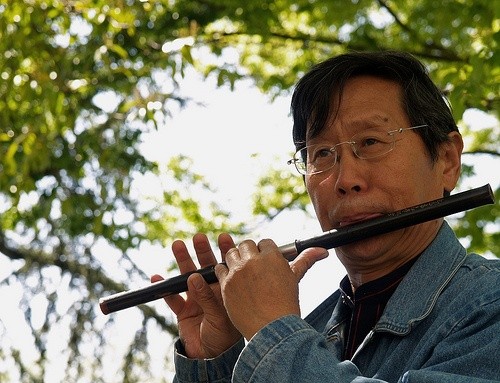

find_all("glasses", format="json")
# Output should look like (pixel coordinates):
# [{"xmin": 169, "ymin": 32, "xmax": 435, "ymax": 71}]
[{"xmin": 287, "ymin": 123, "xmax": 431, "ymax": 176}]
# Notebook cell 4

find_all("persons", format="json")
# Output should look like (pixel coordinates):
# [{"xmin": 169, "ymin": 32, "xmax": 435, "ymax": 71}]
[{"xmin": 150, "ymin": 50, "xmax": 500, "ymax": 383}]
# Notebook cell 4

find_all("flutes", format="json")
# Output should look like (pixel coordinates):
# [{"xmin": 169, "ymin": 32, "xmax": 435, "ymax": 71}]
[{"xmin": 98, "ymin": 184, "xmax": 495, "ymax": 316}]
